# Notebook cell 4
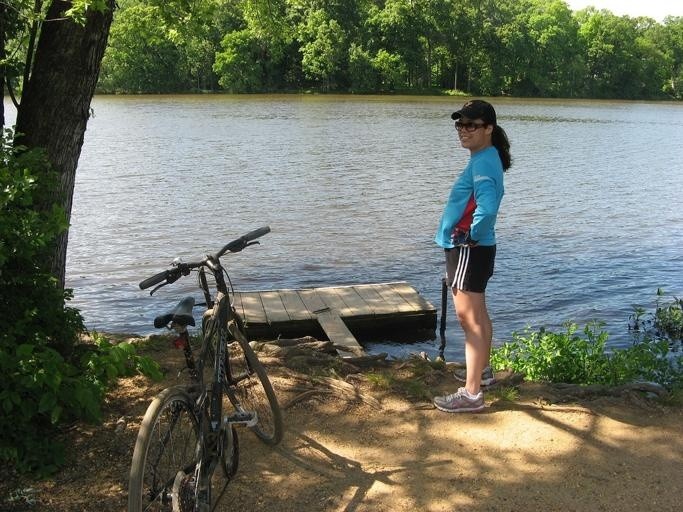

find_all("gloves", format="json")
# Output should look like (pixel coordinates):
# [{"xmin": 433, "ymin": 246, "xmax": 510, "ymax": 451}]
[{"xmin": 451, "ymin": 228, "xmax": 478, "ymax": 248}]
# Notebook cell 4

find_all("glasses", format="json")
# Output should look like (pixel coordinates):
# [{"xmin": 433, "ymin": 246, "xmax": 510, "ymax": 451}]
[{"xmin": 455, "ymin": 121, "xmax": 486, "ymax": 133}]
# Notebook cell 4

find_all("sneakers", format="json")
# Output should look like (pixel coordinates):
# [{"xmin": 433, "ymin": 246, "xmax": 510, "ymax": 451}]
[
  {"xmin": 454, "ymin": 367, "xmax": 496, "ymax": 386},
  {"xmin": 434, "ymin": 387, "xmax": 485, "ymax": 413}
]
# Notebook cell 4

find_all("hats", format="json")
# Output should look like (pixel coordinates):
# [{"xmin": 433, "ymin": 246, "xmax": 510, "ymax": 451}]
[{"xmin": 451, "ymin": 99, "xmax": 496, "ymax": 121}]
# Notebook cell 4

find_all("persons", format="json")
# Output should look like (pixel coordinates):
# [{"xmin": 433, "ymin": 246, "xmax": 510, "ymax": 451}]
[{"xmin": 433, "ymin": 100, "xmax": 513, "ymax": 413}]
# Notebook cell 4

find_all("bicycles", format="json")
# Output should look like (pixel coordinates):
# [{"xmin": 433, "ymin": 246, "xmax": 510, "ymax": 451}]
[{"xmin": 128, "ymin": 225, "xmax": 285, "ymax": 512}]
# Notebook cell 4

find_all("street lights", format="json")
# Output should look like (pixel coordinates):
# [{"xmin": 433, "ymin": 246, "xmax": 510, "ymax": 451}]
[{"xmin": 453, "ymin": 30, "xmax": 458, "ymax": 90}]
